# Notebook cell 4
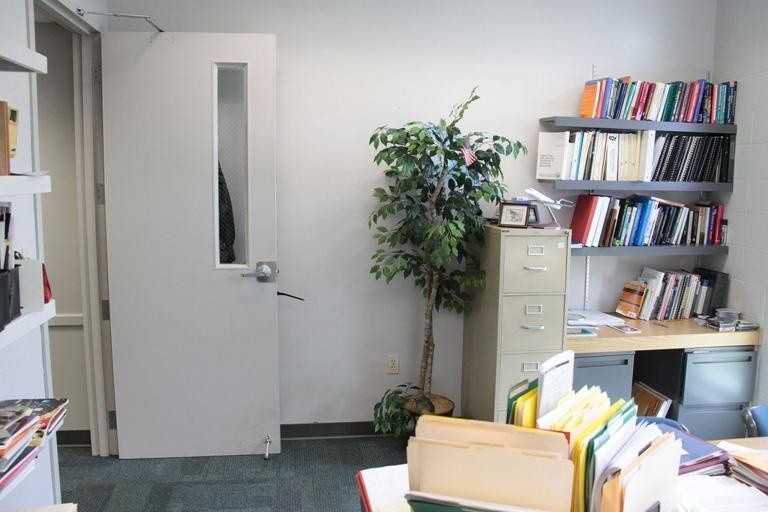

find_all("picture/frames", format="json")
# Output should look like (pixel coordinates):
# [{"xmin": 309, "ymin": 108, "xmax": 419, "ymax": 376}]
[
  {"xmin": 498, "ymin": 202, "xmax": 531, "ymax": 228},
  {"xmin": 514, "ymin": 197, "xmax": 561, "ymax": 230},
  {"xmin": 528, "ymin": 204, "xmax": 541, "ymax": 225}
]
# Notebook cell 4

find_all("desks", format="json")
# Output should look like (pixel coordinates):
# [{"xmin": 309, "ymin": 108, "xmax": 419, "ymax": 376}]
[{"xmin": 354, "ymin": 438, "xmax": 768, "ymax": 512}]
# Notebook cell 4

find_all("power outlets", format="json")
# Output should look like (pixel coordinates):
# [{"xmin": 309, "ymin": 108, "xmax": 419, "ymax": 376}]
[{"xmin": 387, "ymin": 354, "xmax": 399, "ymax": 374}]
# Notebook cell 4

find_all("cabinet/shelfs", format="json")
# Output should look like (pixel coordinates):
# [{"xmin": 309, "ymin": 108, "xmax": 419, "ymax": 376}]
[
  {"xmin": 570, "ymin": 312, "xmax": 762, "ymax": 438},
  {"xmin": 461, "ymin": 217, "xmax": 572, "ymax": 425},
  {"xmin": 0, "ymin": 1, "xmax": 63, "ymax": 509},
  {"xmin": 538, "ymin": 115, "xmax": 761, "ymax": 255}
]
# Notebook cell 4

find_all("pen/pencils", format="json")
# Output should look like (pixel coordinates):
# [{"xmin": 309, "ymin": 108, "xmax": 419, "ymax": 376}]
[{"xmin": 653, "ymin": 322, "xmax": 669, "ymax": 329}]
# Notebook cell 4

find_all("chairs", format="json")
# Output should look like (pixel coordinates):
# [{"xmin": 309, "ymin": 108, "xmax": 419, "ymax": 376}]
[
  {"xmin": 742, "ymin": 403, "xmax": 768, "ymax": 438},
  {"xmin": 637, "ymin": 416, "xmax": 690, "ymax": 435}
]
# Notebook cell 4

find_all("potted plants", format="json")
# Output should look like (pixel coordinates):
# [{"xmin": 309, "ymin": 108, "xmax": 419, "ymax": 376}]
[{"xmin": 366, "ymin": 82, "xmax": 529, "ymax": 445}]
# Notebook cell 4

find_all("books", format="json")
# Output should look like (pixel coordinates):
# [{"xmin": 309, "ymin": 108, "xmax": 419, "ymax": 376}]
[
  {"xmin": 0, "ymin": 396, "xmax": 76, "ymax": 511},
  {"xmin": 537, "ymin": 131, "xmax": 729, "ymax": 183},
  {"xmin": 615, "ymin": 267, "xmax": 729, "ymax": 321},
  {"xmin": 569, "ymin": 194, "xmax": 724, "ymax": 248},
  {"xmin": 406, "ymin": 350, "xmax": 768, "ymax": 512},
  {"xmin": 578, "ymin": 76, "xmax": 736, "ymax": 124}
]
